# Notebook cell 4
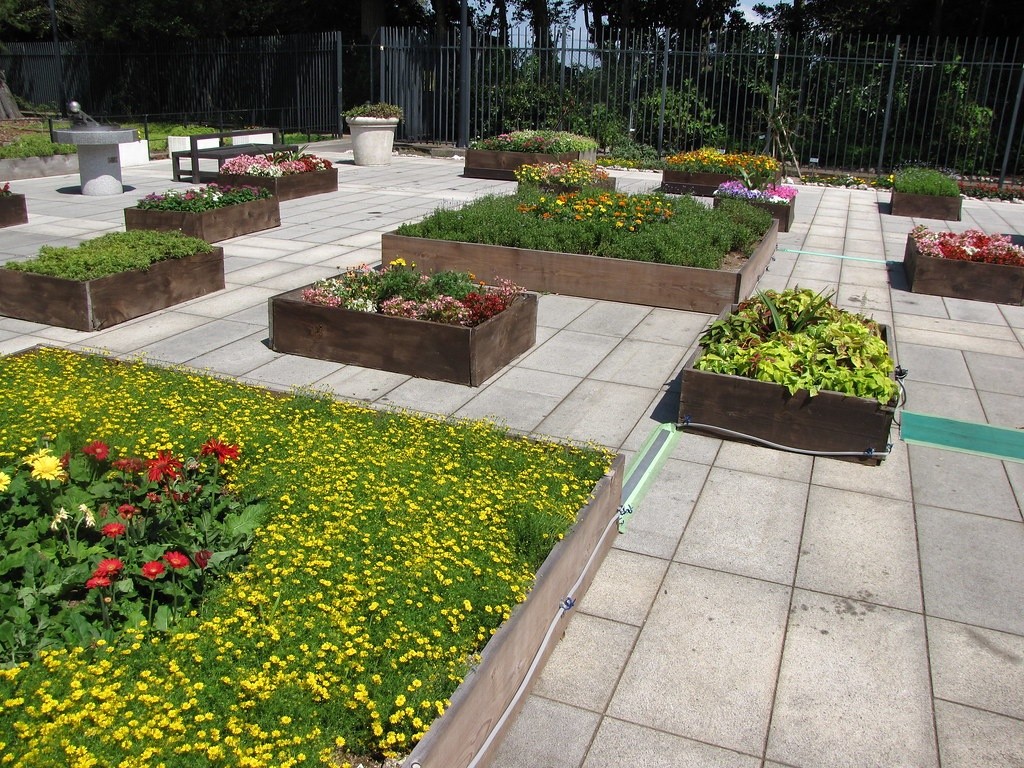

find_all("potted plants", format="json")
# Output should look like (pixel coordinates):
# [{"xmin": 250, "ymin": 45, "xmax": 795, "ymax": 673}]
[
  {"xmin": 0, "ymin": 227, "xmax": 228, "ymax": 330},
  {"xmin": 168, "ymin": 125, "xmax": 220, "ymax": 157},
  {"xmin": 341, "ymin": 102, "xmax": 406, "ymax": 167},
  {"xmin": 890, "ymin": 168, "xmax": 963, "ymax": 223},
  {"xmin": 678, "ymin": 287, "xmax": 898, "ymax": 467},
  {"xmin": 232, "ymin": 127, "xmax": 274, "ymax": 146}
]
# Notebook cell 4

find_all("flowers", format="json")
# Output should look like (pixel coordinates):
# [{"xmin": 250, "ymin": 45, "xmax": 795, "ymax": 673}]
[
  {"xmin": 138, "ymin": 183, "xmax": 271, "ymax": 212},
  {"xmin": 138, "ymin": 130, "xmax": 142, "ymax": 140},
  {"xmin": 514, "ymin": 160, "xmax": 609, "ymax": 190},
  {"xmin": 0, "ymin": 182, "xmax": 12, "ymax": 198},
  {"xmin": 301, "ymin": 259, "xmax": 545, "ymax": 328},
  {"xmin": 470, "ymin": 128, "xmax": 598, "ymax": 156},
  {"xmin": 665, "ymin": 146, "xmax": 780, "ymax": 180},
  {"xmin": 219, "ymin": 144, "xmax": 334, "ymax": 177},
  {"xmin": 714, "ymin": 165, "xmax": 798, "ymax": 206},
  {"xmin": 1, "ymin": 344, "xmax": 620, "ymax": 768},
  {"xmin": 397, "ymin": 182, "xmax": 774, "ymax": 270},
  {"xmin": 911, "ymin": 225, "xmax": 1024, "ymax": 267}
]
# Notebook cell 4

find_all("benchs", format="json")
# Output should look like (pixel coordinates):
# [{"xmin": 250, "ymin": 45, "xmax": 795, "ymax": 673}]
[{"xmin": 171, "ymin": 128, "xmax": 298, "ymax": 186}]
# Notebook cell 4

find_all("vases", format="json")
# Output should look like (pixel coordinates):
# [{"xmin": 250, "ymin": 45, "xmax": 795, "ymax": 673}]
[
  {"xmin": 124, "ymin": 193, "xmax": 281, "ymax": 245},
  {"xmin": 381, "ymin": 215, "xmax": 780, "ymax": 314},
  {"xmin": 220, "ymin": 167, "xmax": 338, "ymax": 204},
  {"xmin": 463, "ymin": 148, "xmax": 598, "ymax": 179},
  {"xmin": 904, "ymin": 234, "xmax": 1024, "ymax": 306},
  {"xmin": 0, "ymin": 193, "xmax": 29, "ymax": 228},
  {"xmin": 267, "ymin": 269, "xmax": 539, "ymax": 386},
  {"xmin": 713, "ymin": 197, "xmax": 796, "ymax": 232},
  {"xmin": 516, "ymin": 177, "xmax": 616, "ymax": 193},
  {"xmin": 1, "ymin": 345, "xmax": 627, "ymax": 768},
  {"xmin": 686, "ymin": 163, "xmax": 784, "ymax": 195},
  {"xmin": 119, "ymin": 140, "xmax": 149, "ymax": 166}
]
